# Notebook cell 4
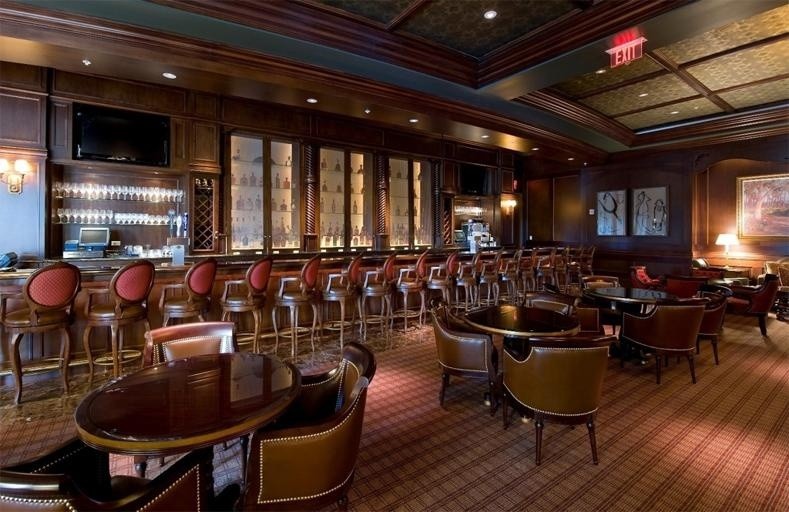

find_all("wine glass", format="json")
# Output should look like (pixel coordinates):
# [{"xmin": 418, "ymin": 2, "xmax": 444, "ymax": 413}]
[
  {"xmin": 57, "ymin": 208, "xmax": 177, "ymax": 225},
  {"xmin": 55, "ymin": 181, "xmax": 183, "ymax": 203}
]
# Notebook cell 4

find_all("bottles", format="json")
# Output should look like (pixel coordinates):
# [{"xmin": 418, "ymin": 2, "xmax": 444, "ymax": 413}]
[
  {"xmin": 321, "ymin": 158, "xmax": 372, "ymax": 246},
  {"xmin": 232, "ymin": 148, "xmax": 300, "ymax": 248},
  {"xmin": 389, "ymin": 165, "xmax": 429, "ymax": 245}
]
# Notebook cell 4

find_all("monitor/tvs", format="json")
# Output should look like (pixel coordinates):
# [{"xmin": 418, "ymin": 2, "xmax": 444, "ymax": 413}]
[
  {"xmin": 71, "ymin": 101, "xmax": 171, "ymax": 168},
  {"xmin": 460, "ymin": 163, "xmax": 489, "ymax": 197},
  {"xmin": 0, "ymin": 252, "xmax": 18, "ymax": 269},
  {"xmin": 79, "ymin": 227, "xmax": 110, "ymax": 247},
  {"xmin": 454, "ymin": 230, "xmax": 466, "ymax": 242}
]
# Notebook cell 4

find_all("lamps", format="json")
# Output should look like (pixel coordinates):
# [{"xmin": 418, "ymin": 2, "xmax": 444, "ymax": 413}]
[{"xmin": 0, "ymin": 155, "xmax": 31, "ymax": 195}]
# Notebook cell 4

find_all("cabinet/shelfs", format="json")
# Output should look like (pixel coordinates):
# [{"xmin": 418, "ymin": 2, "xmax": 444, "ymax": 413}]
[
  {"xmin": 318, "ymin": 140, "xmax": 376, "ymax": 247},
  {"xmin": 223, "ymin": 132, "xmax": 301, "ymax": 256},
  {"xmin": 440, "ymin": 160, "xmax": 496, "ymax": 247},
  {"xmin": 52, "ymin": 183, "xmax": 183, "ymax": 256},
  {"xmin": 387, "ymin": 155, "xmax": 424, "ymax": 249}
]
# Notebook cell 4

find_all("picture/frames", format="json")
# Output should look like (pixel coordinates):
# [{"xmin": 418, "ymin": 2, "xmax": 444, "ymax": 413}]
[{"xmin": 735, "ymin": 172, "xmax": 789, "ymax": 241}]
